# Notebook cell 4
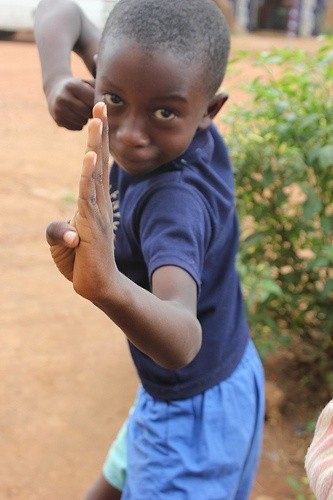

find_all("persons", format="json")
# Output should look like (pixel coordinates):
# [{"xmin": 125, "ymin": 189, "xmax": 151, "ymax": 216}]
[{"xmin": 32, "ymin": 0, "xmax": 269, "ymax": 500}]
